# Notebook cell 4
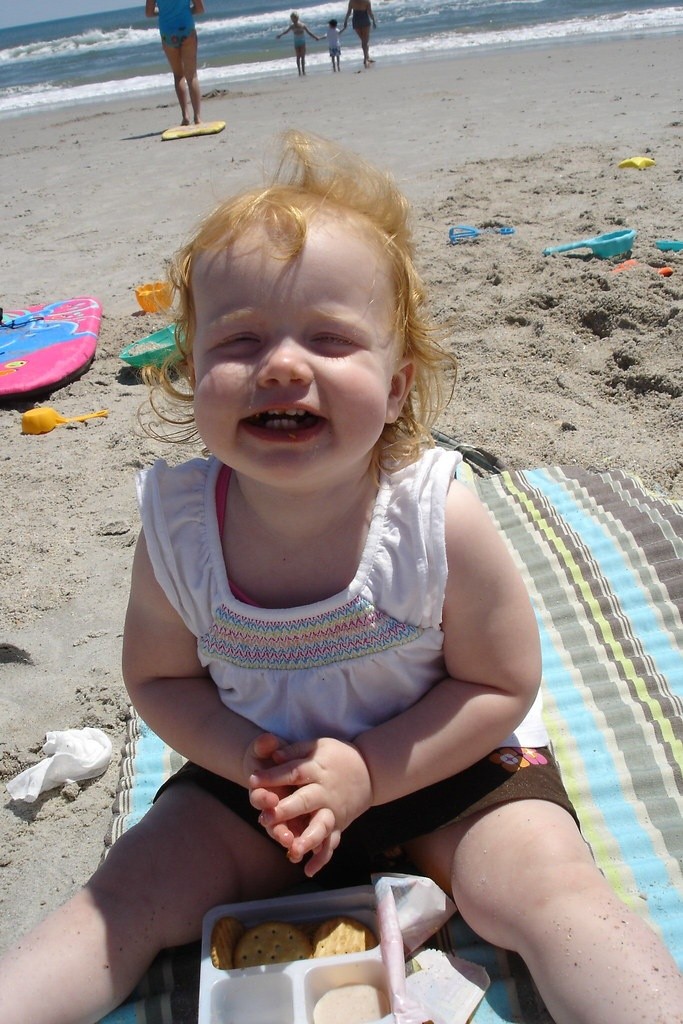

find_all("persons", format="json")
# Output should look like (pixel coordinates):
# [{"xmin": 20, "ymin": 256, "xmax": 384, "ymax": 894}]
[
  {"xmin": 146, "ymin": 0, "xmax": 205, "ymax": 126},
  {"xmin": 317, "ymin": 19, "xmax": 345, "ymax": 72},
  {"xmin": 0, "ymin": 127, "xmax": 683, "ymax": 1023},
  {"xmin": 344, "ymin": 0, "xmax": 376, "ymax": 68},
  {"xmin": 276, "ymin": 12, "xmax": 320, "ymax": 76}
]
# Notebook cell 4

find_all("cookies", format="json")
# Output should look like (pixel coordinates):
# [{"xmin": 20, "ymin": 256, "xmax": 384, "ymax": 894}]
[{"xmin": 211, "ymin": 915, "xmax": 377, "ymax": 970}]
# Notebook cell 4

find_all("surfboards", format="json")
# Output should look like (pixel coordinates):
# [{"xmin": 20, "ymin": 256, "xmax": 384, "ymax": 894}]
[
  {"xmin": 161, "ymin": 121, "xmax": 225, "ymax": 141},
  {"xmin": 0, "ymin": 296, "xmax": 103, "ymax": 402}
]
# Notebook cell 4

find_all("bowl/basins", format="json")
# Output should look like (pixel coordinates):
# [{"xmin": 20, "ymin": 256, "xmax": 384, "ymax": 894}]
[
  {"xmin": 135, "ymin": 283, "xmax": 172, "ymax": 313},
  {"xmin": 655, "ymin": 240, "xmax": 683, "ymax": 252},
  {"xmin": 118, "ymin": 321, "xmax": 187, "ymax": 371}
]
